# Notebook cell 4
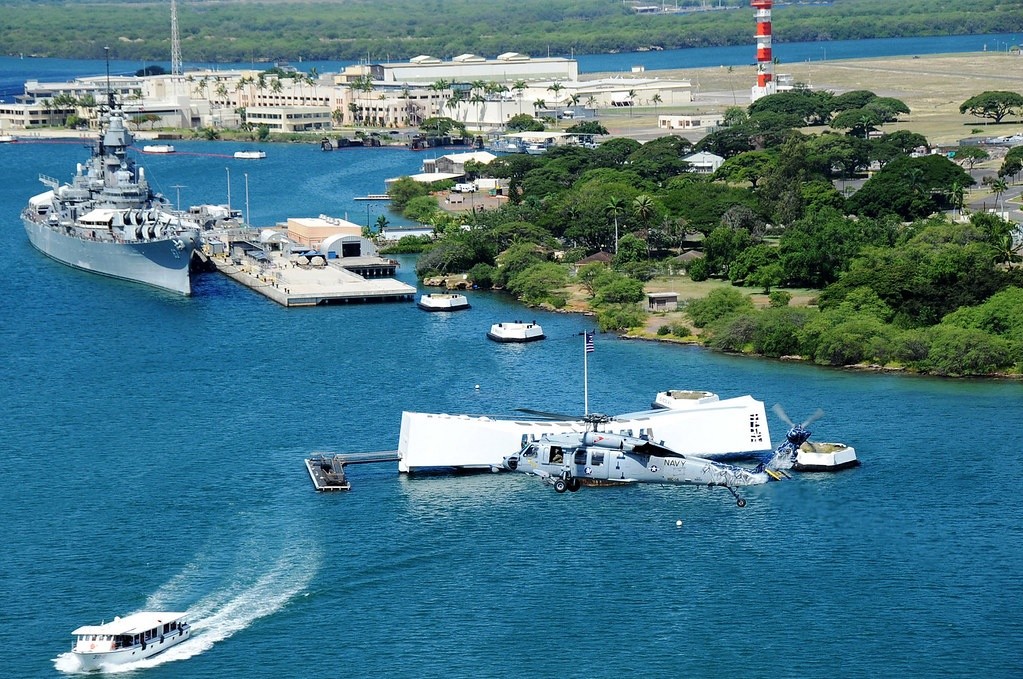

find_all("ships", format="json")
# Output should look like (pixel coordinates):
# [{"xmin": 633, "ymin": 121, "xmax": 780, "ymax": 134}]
[{"xmin": 19, "ymin": 47, "xmax": 200, "ymax": 298}]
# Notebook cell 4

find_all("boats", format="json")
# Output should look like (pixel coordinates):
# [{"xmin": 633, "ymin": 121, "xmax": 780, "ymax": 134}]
[
  {"xmin": 487, "ymin": 130, "xmax": 591, "ymax": 157},
  {"xmin": 69, "ymin": 610, "xmax": 192, "ymax": 672}
]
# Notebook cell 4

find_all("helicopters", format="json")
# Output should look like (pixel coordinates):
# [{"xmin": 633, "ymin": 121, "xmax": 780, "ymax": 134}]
[{"xmin": 501, "ymin": 401, "xmax": 827, "ymax": 509}]
